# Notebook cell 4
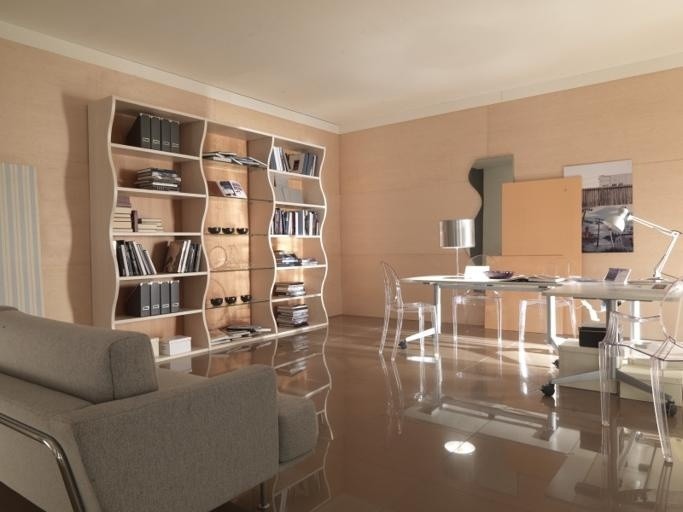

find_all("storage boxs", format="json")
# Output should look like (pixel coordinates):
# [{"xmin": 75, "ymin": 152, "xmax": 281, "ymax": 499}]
[{"xmin": 150, "ymin": 334, "xmax": 191, "ymax": 357}]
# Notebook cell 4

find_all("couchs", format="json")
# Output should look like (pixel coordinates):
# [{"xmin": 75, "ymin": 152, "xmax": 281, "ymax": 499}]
[{"xmin": 0, "ymin": 305, "xmax": 318, "ymax": 512}]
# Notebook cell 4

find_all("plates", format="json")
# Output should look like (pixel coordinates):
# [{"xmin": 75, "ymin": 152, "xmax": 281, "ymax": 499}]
[{"xmin": 482, "ymin": 270, "xmax": 517, "ymax": 278}]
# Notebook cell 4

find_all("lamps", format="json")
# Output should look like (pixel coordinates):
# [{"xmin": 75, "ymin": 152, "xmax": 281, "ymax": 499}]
[
  {"xmin": 438, "ymin": 217, "xmax": 476, "ymax": 276},
  {"xmin": 602, "ymin": 208, "xmax": 682, "ymax": 282}
]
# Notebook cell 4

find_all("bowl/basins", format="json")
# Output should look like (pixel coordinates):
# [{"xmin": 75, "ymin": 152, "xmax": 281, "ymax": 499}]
[
  {"xmin": 236, "ymin": 228, "xmax": 248, "ymax": 234},
  {"xmin": 225, "ymin": 296, "xmax": 236, "ymax": 304},
  {"xmin": 241, "ymin": 294, "xmax": 251, "ymax": 303},
  {"xmin": 222, "ymin": 228, "xmax": 235, "ymax": 234},
  {"xmin": 208, "ymin": 227, "xmax": 221, "ymax": 234},
  {"xmin": 210, "ymin": 298, "xmax": 223, "ymax": 306}
]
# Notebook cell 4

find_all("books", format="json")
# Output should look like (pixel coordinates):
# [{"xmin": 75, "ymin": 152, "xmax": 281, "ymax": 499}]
[
  {"xmin": 211, "ymin": 334, "xmax": 310, "ymax": 376},
  {"xmin": 500, "ymin": 274, "xmax": 566, "ymax": 283},
  {"xmin": 275, "ymin": 471, "xmax": 323, "ymax": 512}
]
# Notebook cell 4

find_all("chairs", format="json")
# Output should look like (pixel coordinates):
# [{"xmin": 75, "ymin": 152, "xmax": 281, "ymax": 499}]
[
  {"xmin": 517, "ymin": 257, "xmax": 576, "ymax": 342},
  {"xmin": 596, "ymin": 277, "xmax": 682, "ymax": 462},
  {"xmin": 379, "ymin": 253, "xmax": 502, "ymax": 362}
]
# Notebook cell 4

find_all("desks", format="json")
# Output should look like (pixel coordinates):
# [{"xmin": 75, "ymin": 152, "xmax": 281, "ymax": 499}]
[{"xmin": 398, "ymin": 274, "xmax": 681, "ymax": 417}]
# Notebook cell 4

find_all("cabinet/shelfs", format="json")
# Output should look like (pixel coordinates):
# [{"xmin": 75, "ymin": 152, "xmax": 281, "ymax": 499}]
[{"xmin": 86, "ymin": 94, "xmax": 329, "ymax": 363}]
